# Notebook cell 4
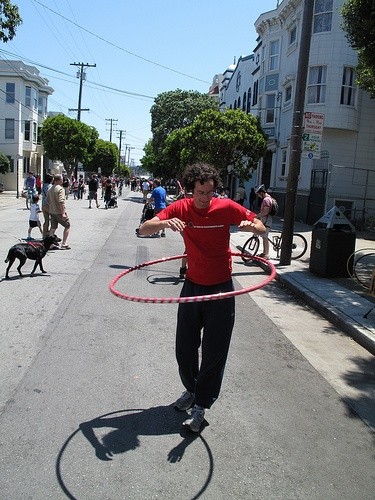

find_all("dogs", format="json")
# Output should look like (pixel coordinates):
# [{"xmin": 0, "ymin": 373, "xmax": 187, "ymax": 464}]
[{"xmin": 4, "ymin": 232, "xmax": 62, "ymax": 278}]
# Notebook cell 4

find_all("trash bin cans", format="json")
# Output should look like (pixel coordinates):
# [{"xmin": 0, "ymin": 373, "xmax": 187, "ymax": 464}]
[{"xmin": 309, "ymin": 206, "xmax": 356, "ymax": 277}]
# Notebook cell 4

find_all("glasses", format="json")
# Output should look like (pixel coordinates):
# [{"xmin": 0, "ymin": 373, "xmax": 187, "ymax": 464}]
[{"xmin": 194, "ymin": 191, "xmax": 216, "ymax": 197}]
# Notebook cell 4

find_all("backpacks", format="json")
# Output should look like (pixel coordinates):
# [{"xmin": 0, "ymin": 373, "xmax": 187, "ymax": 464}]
[{"xmin": 265, "ymin": 196, "xmax": 279, "ymax": 217}]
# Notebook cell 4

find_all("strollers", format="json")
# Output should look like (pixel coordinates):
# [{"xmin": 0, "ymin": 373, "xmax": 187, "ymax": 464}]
[
  {"xmin": 136, "ymin": 203, "xmax": 158, "ymax": 237},
  {"xmin": 108, "ymin": 191, "xmax": 119, "ymax": 207}
]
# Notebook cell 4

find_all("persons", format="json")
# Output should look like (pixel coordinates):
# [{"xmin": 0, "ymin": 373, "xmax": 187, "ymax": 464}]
[
  {"xmin": 101, "ymin": 174, "xmax": 123, "ymax": 198},
  {"xmin": 28, "ymin": 195, "xmax": 42, "ymax": 238},
  {"xmin": 88, "ymin": 174, "xmax": 100, "ymax": 208},
  {"xmin": 63, "ymin": 174, "xmax": 85, "ymax": 199},
  {"xmin": 138, "ymin": 162, "xmax": 266, "ymax": 433},
  {"xmin": 124, "ymin": 176, "xmax": 153, "ymax": 193},
  {"xmin": 45, "ymin": 174, "xmax": 71, "ymax": 249},
  {"xmin": 142, "ymin": 200, "xmax": 152, "ymax": 212},
  {"xmin": 42, "ymin": 174, "xmax": 59, "ymax": 242},
  {"xmin": 146, "ymin": 179, "xmax": 167, "ymax": 236},
  {"xmin": 248, "ymin": 188, "xmax": 255, "ymax": 212},
  {"xmin": 104, "ymin": 179, "xmax": 113, "ymax": 209},
  {"xmin": 178, "ymin": 249, "xmax": 187, "ymax": 278},
  {"xmin": 254, "ymin": 184, "xmax": 274, "ymax": 259},
  {"xmin": 25, "ymin": 171, "xmax": 42, "ymax": 202},
  {"xmin": 218, "ymin": 184, "xmax": 247, "ymax": 206},
  {"xmin": 86, "ymin": 173, "xmax": 101, "ymax": 188}
]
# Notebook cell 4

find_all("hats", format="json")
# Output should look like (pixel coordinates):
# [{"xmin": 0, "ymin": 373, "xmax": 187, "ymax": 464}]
[{"xmin": 106, "ymin": 179, "xmax": 112, "ymax": 183}]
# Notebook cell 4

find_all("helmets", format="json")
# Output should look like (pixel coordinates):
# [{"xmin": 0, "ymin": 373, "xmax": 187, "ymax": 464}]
[{"xmin": 254, "ymin": 184, "xmax": 265, "ymax": 194}]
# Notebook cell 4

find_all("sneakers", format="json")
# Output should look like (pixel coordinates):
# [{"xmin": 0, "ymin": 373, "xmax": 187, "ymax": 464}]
[
  {"xmin": 173, "ymin": 390, "xmax": 195, "ymax": 410},
  {"xmin": 181, "ymin": 405, "xmax": 205, "ymax": 432}
]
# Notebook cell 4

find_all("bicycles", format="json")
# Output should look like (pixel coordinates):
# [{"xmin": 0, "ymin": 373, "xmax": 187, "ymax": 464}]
[
  {"xmin": 354, "ymin": 253, "xmax": 375, "ymax": 318},
  {"xmin": 241, "ymin": 218, "xmax": 308, "ymax": 261},
  {"xmin": 23, "ymin": 187, "xmax": 33, "ymax": 210}
]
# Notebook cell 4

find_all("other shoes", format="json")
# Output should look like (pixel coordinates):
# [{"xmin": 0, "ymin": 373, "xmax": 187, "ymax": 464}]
[
  {"xmin": 179, "ymin": 266, "xmax": 187, "ymax": 278},
  {"xmin": 152, "ymin": 233, "xmax": 166, "ymax": 238},
  {"xmin": 61, "ymin": 244, "xmax": 71, "ymax": 250},
  {"xmin": 27, "ymin": 237, "xmax": 35, "ymax": 240},
  {"xmin": 259, "ymin": 253, "xmax": 271, "ymax": 260}
]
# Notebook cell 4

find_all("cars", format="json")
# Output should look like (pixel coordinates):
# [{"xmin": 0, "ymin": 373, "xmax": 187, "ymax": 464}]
[{"xmin": 0, "ymin": 183, "xmax": 5, "ymax": 193}]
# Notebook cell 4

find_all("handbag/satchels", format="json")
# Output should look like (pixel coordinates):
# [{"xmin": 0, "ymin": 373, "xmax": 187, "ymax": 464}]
[{"xmin": 145, "ymin": 206, "xmax": 154, "ymax": 220}]
